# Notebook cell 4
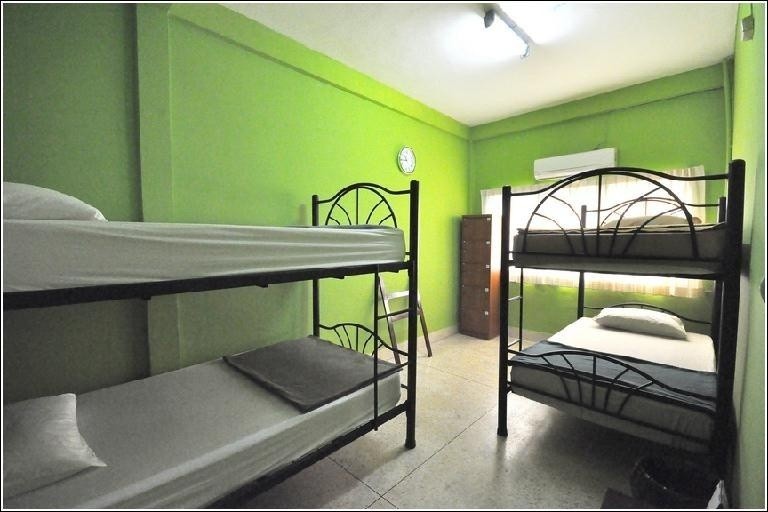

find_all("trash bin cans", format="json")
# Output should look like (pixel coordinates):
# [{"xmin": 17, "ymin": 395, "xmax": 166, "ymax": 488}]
[{"xmin": 632, "ymin": 455, "xmax": 718, "ymax": 508}]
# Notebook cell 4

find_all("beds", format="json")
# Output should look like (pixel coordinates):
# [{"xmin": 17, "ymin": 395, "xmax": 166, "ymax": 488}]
[
  {"xmin": 497, "ymin": 159, "xmax": 746, "ymax": 481},
  {"xmin": 3, "ymin": 179, "xmax": 420, "ymax": 509}
]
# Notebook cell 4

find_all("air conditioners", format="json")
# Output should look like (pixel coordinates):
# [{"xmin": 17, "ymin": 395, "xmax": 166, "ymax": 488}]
[{"xmin": 534, "ymin": 147, "xmax": 617, "ymax": 181}]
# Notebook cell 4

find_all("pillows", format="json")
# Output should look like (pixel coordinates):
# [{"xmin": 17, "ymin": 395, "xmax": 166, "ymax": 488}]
[
  {"xmin": 4, "ymin": 181, "xmax": 108, "ymax": 222},
  {"xmin": 603, "ymin": 217, "xmax": 702, "ymax": 228},
  {"xmin": 3, "ymin": 393, "xmax": 108, "ymax": 498},
  {"xmin": 592, "ymin": 307, "xmax": 687, "ymax": 339}
]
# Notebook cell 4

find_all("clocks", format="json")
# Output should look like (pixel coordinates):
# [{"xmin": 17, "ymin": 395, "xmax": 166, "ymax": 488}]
[{"xmin": 397, "ymin": 146, "xmax": 416, "ymax": 175}]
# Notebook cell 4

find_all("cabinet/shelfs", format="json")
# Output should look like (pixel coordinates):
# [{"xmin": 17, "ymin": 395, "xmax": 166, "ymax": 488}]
[{"xmin": 460, "ymin": 214, "xmax": 501, "ymax": 340}]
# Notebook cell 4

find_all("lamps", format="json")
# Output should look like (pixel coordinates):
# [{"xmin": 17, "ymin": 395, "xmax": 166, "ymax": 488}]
[{"xmin": 483, "ymin": 3, "xmax": 537, "ymax": 62}]
